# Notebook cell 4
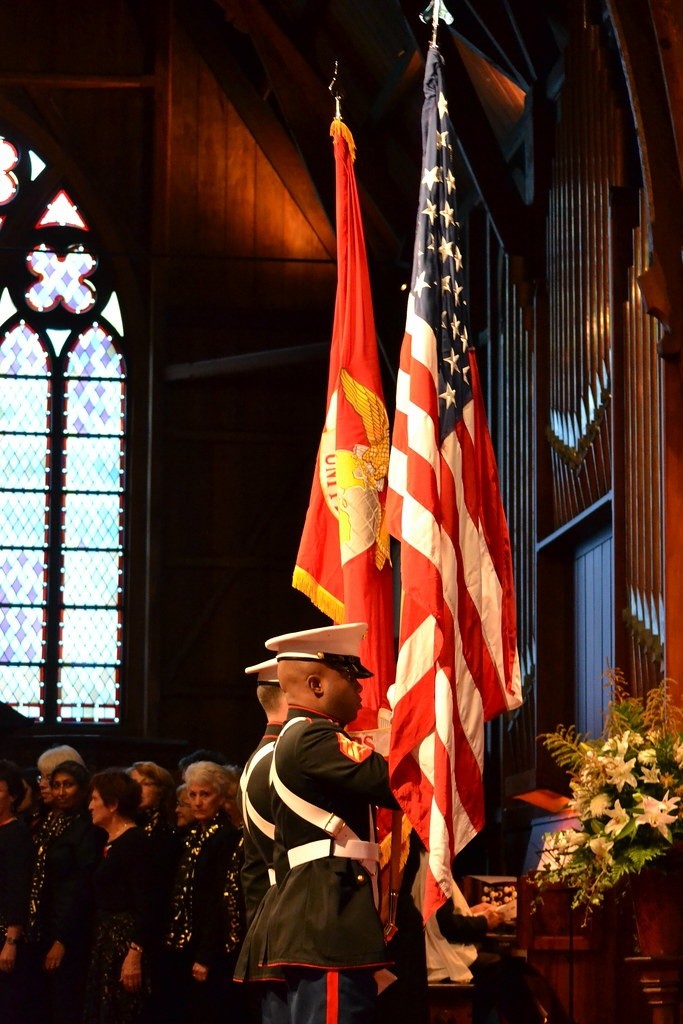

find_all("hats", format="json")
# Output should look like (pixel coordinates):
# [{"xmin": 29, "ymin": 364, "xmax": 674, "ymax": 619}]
[
  {"xmin": 265, "ymin": 620, "xmax": 375, "ymax": 680},
  {"xmin": 246, "ymin": 653, "xmax": 283, "ymax": 690}
]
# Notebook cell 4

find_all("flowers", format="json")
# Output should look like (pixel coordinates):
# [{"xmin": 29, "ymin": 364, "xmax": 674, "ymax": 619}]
[{"xmin": 528, "ymin": 663, "xmax": 683, "ymax": 927}]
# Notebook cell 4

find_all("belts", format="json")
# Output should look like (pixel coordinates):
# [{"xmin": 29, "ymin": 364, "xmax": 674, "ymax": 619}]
[
  {"xmin": 267, "ymin": 867, "xmax": 280, "ymax": 888},
  {"xmin": 275, "ymin": 839, "xmax": 380, "ymax": 869}
]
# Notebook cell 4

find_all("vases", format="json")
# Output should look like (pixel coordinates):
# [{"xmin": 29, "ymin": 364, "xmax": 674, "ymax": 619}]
[{"xmin": 629, "ymin": 868, "xmax": 683, "ymax": 960}]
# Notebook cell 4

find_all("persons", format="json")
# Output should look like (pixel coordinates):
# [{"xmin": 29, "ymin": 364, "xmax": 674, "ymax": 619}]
[{"xmin": 0, "ymin": 621, "xmax": 525, "ymax": 1024}]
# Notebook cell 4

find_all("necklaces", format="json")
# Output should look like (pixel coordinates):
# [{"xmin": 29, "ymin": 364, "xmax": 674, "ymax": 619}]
[{"xmin": 107, "ymin": 821, "xmax": 129, "ymax": 842}]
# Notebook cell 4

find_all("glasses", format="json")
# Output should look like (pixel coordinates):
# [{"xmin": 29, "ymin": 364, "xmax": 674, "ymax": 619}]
[{"xmin": 36, "ymin": 775, "xmax": 52, "ymax": 784}]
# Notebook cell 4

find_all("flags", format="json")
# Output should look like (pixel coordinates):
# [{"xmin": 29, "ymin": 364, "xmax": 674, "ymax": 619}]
[
  {"xmin": 292, "ymin": 120, "xmax": 414, "ymax": 927},
  {"xmin": 516, "ymin": 789, "xmax": 569, "ymax": 813},
  {"xmin": 380, "ymin": 45, "xmax": 523, "ymax": 923}
]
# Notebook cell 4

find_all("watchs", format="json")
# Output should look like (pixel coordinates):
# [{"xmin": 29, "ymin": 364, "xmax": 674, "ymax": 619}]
[
  {"xmin": 4, "ymin": 936, "xmax": 18, "ymax": 944},
  {"xmin": 128, "ymin": 944, "xmax": 143, "ymax": 952}
]
